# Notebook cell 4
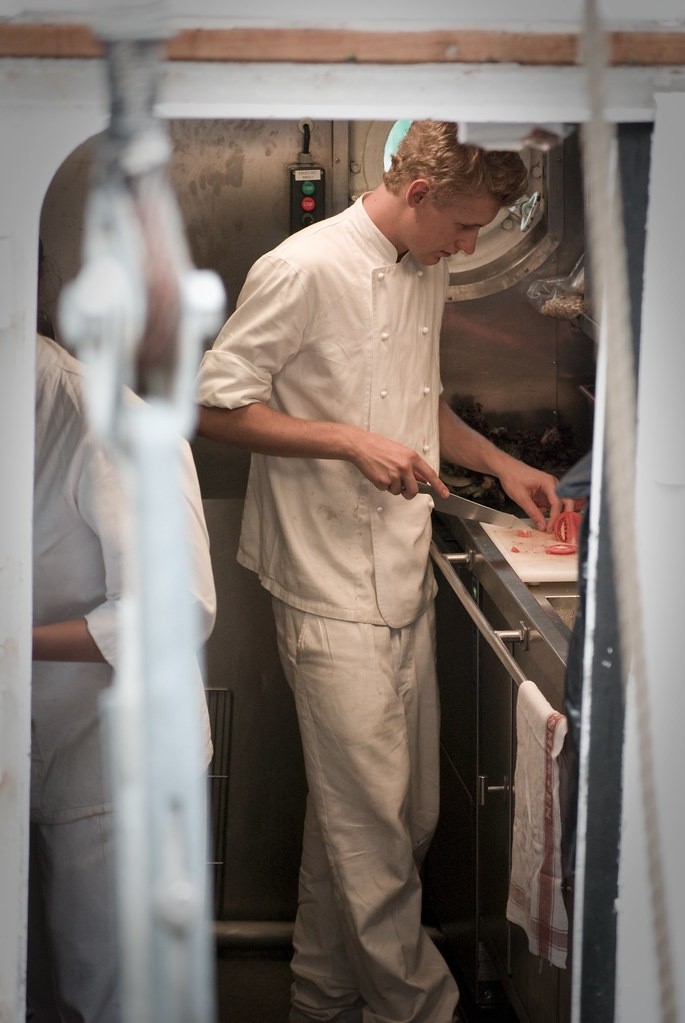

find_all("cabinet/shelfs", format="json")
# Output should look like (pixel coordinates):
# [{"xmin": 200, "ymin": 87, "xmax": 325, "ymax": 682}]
[{"xmin": 421, "ymin": 537, "xmax": 580, "ymax": 1023}]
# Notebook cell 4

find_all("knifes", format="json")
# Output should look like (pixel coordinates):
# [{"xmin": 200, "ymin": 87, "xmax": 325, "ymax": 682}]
[{"xmin": 414, "ymin": 480, "xmax": 530, "ymax": 532}]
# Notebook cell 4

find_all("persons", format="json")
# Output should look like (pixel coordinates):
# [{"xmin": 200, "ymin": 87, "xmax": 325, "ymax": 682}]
[
  {"xmin": 32, "ymin": 335, "xmax": 215, "ymax": 1023},
  {"xmin": 191, "ymin": 124, "xmax": 581, "ymax": 1023}
]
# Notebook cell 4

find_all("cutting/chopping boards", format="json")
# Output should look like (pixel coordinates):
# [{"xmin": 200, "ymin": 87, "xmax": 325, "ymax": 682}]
[{"xmin": 478, "ymin": 517, "xmax": 580, "ymax": 585}]
